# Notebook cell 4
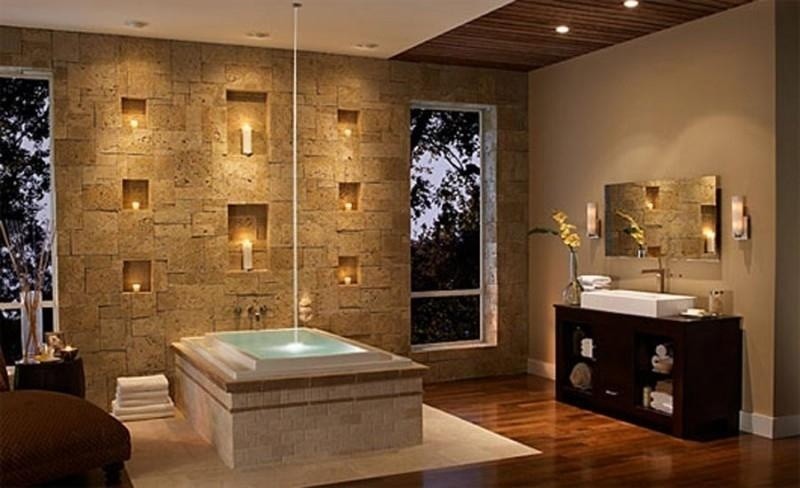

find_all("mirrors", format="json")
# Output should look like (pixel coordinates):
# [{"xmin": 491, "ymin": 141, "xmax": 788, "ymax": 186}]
[{"xmin": 604, "ymin": 175, "xmax": 721, "ymax": 262}]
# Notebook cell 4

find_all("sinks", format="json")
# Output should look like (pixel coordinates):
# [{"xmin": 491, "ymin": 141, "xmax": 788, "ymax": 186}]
[{"xmin": 579, "ymin": 288, "xmax": 695, "ymax": 319}]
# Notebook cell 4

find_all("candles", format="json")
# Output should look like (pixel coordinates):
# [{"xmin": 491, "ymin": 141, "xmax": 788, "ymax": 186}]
[
  {"xmin": 241, "ymin": 240, "xmax": 253, "ymax": 271},
  {"xmin": 242, "ymin": 126, "xmax": 253, "ymax": 154}
]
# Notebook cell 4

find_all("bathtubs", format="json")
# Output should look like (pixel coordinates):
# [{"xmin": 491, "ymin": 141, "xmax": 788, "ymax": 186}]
[{"xmin": 179, "ymin": 325, "xmax": 414, "ymax": 383}]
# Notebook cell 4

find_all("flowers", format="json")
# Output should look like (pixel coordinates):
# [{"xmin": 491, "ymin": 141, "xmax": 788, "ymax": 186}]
[
  {"xmin": 528, "ymin": 207, "xmax": 584, "ymax": 252},
  {"xmin": 613, "ymin": 211, "xmax": 651, "ymax": 250}
]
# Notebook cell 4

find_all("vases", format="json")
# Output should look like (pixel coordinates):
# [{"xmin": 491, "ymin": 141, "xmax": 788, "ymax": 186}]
[
  {"xmin": 561, "ymin": 253, "xmax": 585, "ymax": 304},
  {"xmin": 19, "ymin": 290, "xmax": 45, "ymax": 364}
]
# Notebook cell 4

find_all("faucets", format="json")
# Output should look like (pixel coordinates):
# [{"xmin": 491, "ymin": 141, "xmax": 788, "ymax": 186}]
[{"xmin": 641, "ymin": 257, "xmax": 665, "ymax": 292}]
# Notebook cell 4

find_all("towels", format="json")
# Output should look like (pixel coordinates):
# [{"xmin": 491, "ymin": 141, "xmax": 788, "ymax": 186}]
[
  {"xmin": 577, "ymin": 272, "xmax": 612, "ymax": 292},
  {"xmin": 649, "ymin": 390, "xmax": 674, "ymax": 415},
  {"xmin": 579, "ymin": 337, "xmax": 593, "ymax": 358},
  {"xmin": 110, "ymin": 372, "xmax": 177, "ymax": 422}
]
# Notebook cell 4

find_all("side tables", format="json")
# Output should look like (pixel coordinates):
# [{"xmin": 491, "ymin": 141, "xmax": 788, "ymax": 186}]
[{"xmin": 14, "ymin": 356, "xmax": 86, "ymax": 400}]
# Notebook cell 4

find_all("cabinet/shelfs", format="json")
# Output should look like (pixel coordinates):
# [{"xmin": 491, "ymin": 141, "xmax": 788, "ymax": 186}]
[{"xmin": 552, "ymin": 304, "xmax": 743, "ymax": 443}]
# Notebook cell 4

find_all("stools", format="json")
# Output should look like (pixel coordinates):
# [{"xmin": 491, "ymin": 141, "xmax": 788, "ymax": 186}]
[{"xmin": 1, "ymin": 388, "xmax": 132, "ymax": 486}]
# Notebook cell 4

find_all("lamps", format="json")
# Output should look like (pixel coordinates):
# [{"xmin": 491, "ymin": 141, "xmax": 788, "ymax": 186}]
[
  {"xmin": 583, "ymin": 201, "xmax": 603, "ymax": 240},
  {"xmin": 731, "ymin": 193, "xmax": 751, "ymax": 242}
]
